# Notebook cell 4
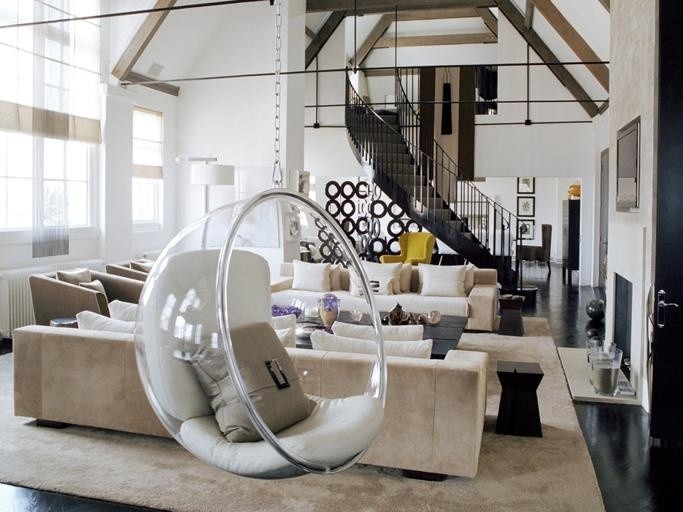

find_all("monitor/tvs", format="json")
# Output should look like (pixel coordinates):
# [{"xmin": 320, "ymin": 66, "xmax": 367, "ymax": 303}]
[{"xmin": 616, "ymin": 115, "xmax": 641, "ymax": 213}]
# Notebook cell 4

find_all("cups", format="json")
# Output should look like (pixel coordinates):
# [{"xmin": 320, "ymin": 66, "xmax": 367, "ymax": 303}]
[
  {"xmin": 583, "ymin": 337, "xmax": 623, "ymax": 394},
  {"xmin": 350, "ymin": 305, "xmax": 363, "ymax": 321},
  {"xmin": 427, "ymin": 310, "xmax": 441, "ymax": 325}
]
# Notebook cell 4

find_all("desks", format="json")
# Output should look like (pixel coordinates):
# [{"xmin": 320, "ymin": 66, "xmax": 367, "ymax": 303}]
[
  {"xmin": 492, "ymin": 358, "xmax": 544, "ymax": 440},
  {"xmin": 496, "ymin": 295, "xmax": 527, "ymax": 337}
]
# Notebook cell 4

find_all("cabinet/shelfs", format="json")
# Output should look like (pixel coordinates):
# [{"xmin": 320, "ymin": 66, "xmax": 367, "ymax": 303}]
[{"xmin": 560, "ymin": 199, "xmax": 580, "ymax": 288}]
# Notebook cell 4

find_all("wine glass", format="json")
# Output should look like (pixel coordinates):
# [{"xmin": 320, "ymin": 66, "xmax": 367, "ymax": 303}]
[{"xmin": 320, "ymin": 297, "xmax": 341, "ymax": 334}]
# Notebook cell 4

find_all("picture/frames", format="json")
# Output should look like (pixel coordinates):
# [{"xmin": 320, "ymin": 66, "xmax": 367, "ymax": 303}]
[{"xmin": 517, "ymin": 176, "xmax": 535, "ymax": 241}]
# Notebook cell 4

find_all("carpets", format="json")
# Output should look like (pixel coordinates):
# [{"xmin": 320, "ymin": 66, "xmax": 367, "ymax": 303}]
[{"xmin": 0, "ymin": 317, "xmax": 609, "ymax": 511}]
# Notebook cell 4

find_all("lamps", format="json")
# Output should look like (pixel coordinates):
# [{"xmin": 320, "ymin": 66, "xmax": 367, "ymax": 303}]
[{"xmin": 175, "ymin": 153, "xmax": 238, "ymax": 249}]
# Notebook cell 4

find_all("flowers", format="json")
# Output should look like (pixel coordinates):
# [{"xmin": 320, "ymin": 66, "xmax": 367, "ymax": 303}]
[{"xmin": 271, "ymin": 303, "xmax": 302, "ymax": 316}]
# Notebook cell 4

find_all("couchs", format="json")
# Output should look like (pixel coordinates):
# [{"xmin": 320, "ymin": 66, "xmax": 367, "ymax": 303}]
[
  {"xmin": 12, "ymin": 320, "xmax": 490, "ymax": 480},
  {"xmin": 270, "ymin": 261, "xmax": 498, "ymax": 332},
  {"xmin": 30, "ymin": 267, "xmax": 145, "ymax": 325},
  {"xmin": 106, "ymin": 259, "xmax": 152, "ymax": 282}
]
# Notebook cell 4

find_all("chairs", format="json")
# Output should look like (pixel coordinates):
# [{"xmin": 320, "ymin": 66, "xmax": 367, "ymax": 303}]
[
  {"xmin": 376, "ymin": 232, "xmax": 436, "ymax": 265},
  {"xmin": 515, "ymin": 222, "xmax": 552, "ymax": 277}
]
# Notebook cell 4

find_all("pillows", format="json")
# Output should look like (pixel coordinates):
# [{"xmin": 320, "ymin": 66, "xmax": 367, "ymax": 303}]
[
  {"xmin": 108, "ymin": 298, "xmax": 139, "ymax": 322},
  {"xmin": 56, "ymin": 269, "xmax": 92, "ymax": 286},
  {"xmin": 129, "ymin": 260, "xmax": 152, "ymax": 272},
  {"xmin": 75, "ymin": 309, "xmax": 136, "ymax": 334},
  {"xmin": 308, "ymin": 328, "xmax": 434, "ymax": 359},
  {"xmin": 418, "ymin": 261, "xmax": 476, "ymax": 296},
  {"xmin": 348, "ymin": 262, "xmax": 402, "ymax": 295},
  {"xmin": 79, "ymin": 279, "xmax": 109, "ymax": 305},
  {"xmin": 290, "ymin": 259, "xmax": 331, "ymax": 292},
  {"xmin": 402, "ymin": 264, "xmax": 413, "ymax": 293},
  {"xmin": 331, "ymin": 320, "xmax": 424, "ymax": 343},
  {"xmin": 275, "ymin": 327, "xmax": 297, "ymax": 348},
  {"xmin": 180, "ymin": 316, "xmax": 320, "ymax": 442},
  {"xmin": 270, "ymin": 313, "xmax": 297, "ymax": 331},
  {"xmin": 329, "ymin": 263, "xmax": 342, "ymax": 291},
  {"xmin": 349, "ymin": 277, "xmax": 396, "ymax": 295},
  {"xmin": 420, "ymin": 266, "xmax": 467, "ymax": 297}
]
